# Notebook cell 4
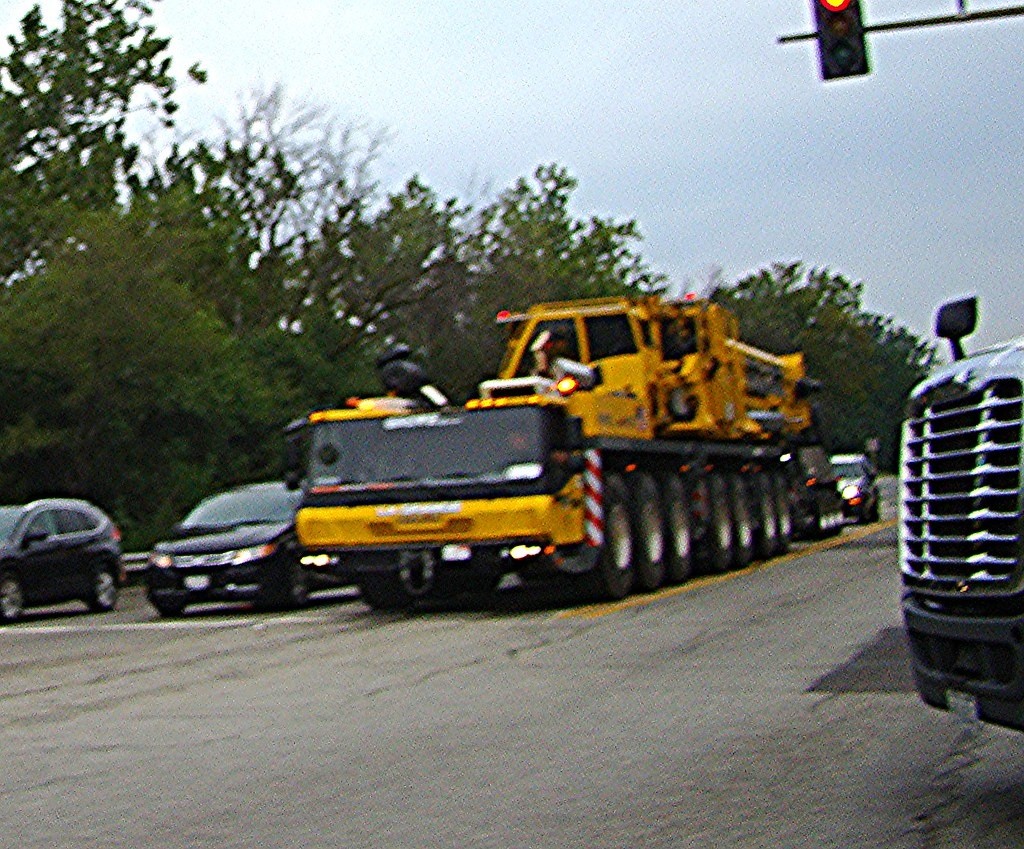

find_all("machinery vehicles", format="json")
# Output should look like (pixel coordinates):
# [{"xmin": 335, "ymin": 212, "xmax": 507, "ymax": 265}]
[{"xmin": 281, "ymin": 290, "xmax": 844, "ymax": 610}]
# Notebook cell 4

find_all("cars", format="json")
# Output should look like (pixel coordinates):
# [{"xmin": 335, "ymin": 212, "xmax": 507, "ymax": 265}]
[
  {"xmin": 895, "ymin": 296, "xmax": 1023, "ymax": 733},
  {"xmin": 0, "ymin": 497, "xmax": 126, "ymax": 626},
  {"xmin": 829, "ymin": 454, "xmax": 881, "ymax": 525},
  {"xmin": 141, "ymin": 481, "xmax": 353, "ymax": 617}
]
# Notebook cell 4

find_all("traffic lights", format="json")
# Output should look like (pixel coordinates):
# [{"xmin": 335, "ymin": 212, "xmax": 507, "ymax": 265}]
[{"xmin": 811, "ymin": 0, "xmax": 870, "ymax": 82}]
